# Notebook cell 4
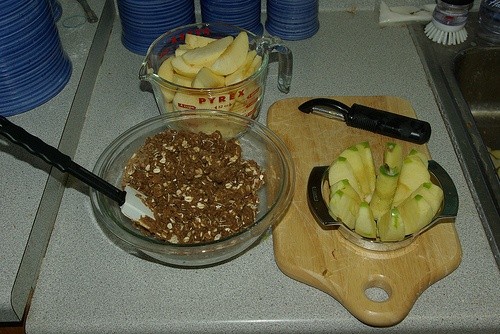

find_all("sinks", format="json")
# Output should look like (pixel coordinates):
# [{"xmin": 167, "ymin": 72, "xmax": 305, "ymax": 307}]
[{"xmin": 450, "ymin": 47, "xmax": 499, "ymax": 183}]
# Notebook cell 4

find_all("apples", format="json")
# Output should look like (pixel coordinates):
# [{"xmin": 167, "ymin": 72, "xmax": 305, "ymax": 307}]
[
  {"xmin": 322, "ymin": 141, "xmax": 444, "ymax": 242},
  {"xmin": 157, "ymin": 33, "xmax": 264, "ymax": 139}
]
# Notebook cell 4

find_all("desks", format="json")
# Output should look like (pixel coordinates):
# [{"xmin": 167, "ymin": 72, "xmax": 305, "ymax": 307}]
[{"xmin": 0, "ymin": 0, "xmax": 500, "ymax": 334}]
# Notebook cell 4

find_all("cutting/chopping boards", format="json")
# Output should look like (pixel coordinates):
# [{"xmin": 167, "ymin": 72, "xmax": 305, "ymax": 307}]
[{"xmin": 266, "ymin": 96, "xmax": 463, "ymax": 328}]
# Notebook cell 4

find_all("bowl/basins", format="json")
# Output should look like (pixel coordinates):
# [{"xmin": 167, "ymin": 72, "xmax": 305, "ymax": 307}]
[
  {"xmin": 87, "ymin": 109, "xmax": 296, "ymax": 269},
  {"xmin": 138, "ymin": 21, "xmax": 293, "ymax": 140}
]
